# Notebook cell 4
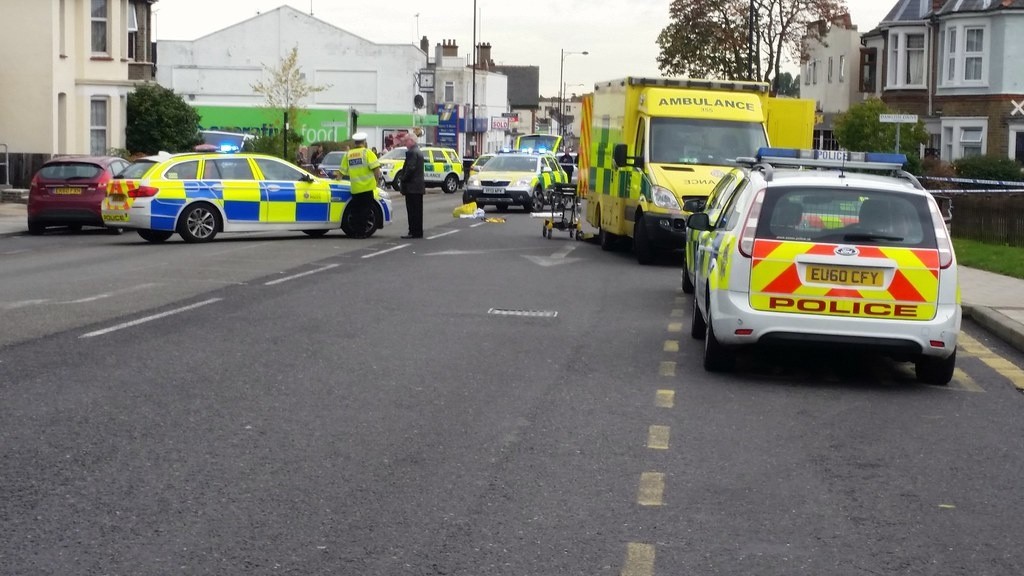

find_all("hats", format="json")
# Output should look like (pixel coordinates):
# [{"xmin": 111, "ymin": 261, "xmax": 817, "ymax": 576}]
[
  {"xmin": 352, "ymin": 132, "xmax": 367, "ymax": 141},
  {"xmin": 401, "ymin": 133, "xmax": 418, "ymax": 142}
]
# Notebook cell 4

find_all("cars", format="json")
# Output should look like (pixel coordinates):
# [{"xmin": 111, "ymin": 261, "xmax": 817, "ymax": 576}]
[
  {"xmin": 27, "ymin": 145, "xmax": 393, "ymax": 243},
  {"xmin": 682, "ymin": 148, "xmax": 962, "ymax": 385},
  {"xmin": 318, "ymin": 151, "xmax": 350, "ymax": 180}
]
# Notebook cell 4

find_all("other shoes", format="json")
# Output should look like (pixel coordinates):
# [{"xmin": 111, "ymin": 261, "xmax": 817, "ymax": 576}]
[{"xmin": 401, "ymin": 234, "xmax": 423, "ymax": 239}]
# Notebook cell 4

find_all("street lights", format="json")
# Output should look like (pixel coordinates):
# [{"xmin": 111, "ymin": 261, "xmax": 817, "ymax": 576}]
[{"xmin": 558, "ymin": 49, "xmax": 589, "ymax": 135}]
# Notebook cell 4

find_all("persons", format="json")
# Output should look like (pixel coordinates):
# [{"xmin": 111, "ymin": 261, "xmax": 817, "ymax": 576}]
[
  {"xmin": 559, "ymin": 147, "xmax": 574, "ymax": 184},
  {"xmin": 462, "ymin": 149, "xmax": 473, "ymax": 186},
  {"xmin": 336, "ymin": 132, "xmax": 382, "ymax": 238},
  {"xmin": 400, "ymin": 133, "xmax": 426, "ymax": 239},
  {"xmin": 311, "ymin": 145, "xmax": 324, "ymax": 174}
]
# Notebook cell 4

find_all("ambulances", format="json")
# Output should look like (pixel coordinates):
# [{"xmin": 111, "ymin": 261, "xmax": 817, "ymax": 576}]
[
  {"xmin": 378, "ymin": 143, "xmax": 464, "ymax": 194},
  {"xmin": 463, "ymin": 134, "xmax": 569, "ymax": 213},
  {"xmin": 577, "ymin": 76, "xmax": 815, "ymax": 264}
]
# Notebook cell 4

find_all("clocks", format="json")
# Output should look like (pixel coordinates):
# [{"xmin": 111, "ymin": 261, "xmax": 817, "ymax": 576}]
[{"xmin": 419, "ymin": 68, "xmax": 435, "ymax": 93}]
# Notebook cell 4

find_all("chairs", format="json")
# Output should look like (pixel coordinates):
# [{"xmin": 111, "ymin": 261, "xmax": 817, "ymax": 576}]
[
  {"xmin": 64, "ymin": 166, "xmax": 76, "ymax": 175},
  {"xmin": 771, "ymin": 203, "xmax": 803, "ymax": 225},
  {"xmin": 721, "ymin": 134, "xmax": 738, "ymax": 148},
  {"xmin": 859, "ymin": 200, "xmax": 890, "ymax": 224}
]
